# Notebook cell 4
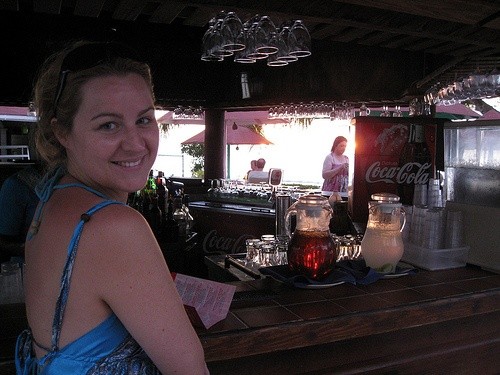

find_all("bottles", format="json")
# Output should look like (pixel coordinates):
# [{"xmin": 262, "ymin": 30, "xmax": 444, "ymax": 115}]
[
  {"xmin": 126, "ymin": 169, "xmax": 194, "ymax": 241},
  {"xmin": 0, "ymin": 256, "xmax": 25, "ymax": 298},
  {"xmin": 397, "ymin": 121, "xmax": 422, "ymax": 205}
]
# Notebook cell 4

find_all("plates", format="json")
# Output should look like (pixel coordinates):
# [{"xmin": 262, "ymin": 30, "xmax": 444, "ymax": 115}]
[
  {"xmin": 378, "ymin": 262, "xmax": 414, "ymax": 280},
  {"xmin": 292, "ymin": 280, "xmax": 346, "ymax": 288}
]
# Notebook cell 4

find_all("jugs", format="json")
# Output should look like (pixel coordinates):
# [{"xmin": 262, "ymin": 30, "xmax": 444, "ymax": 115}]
[
  {"xmin": 283, "ymin": 194, "xmax": 338, "ymax": 281},
  {"xmin": 361, "ymin": 192, "xmax": 407, "ymax": 273}
]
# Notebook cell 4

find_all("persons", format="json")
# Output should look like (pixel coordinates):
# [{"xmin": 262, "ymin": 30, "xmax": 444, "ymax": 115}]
[
  {"xmin": 0, "ymin": 163, "xmax": 43, "ymax": 253},
  {"xmin": 247, "ymin": 158, "xmax": 265, "ymax": 180},
  {"xmin": 322, "ymin": 136, "xmax": 349, "ymax": 192},
  {"xmin": 16, "ymin": 40, "xmax": 212, "ymax": 375}
]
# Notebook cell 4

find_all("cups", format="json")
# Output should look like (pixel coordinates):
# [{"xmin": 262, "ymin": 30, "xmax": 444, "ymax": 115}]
[
  {"xmin": 399, "ymin": 178, "xmax": 464, "ymax": 250},
  {"xmin": 246, "ymin": 233, "xmax": 364, "ymax": 267}
]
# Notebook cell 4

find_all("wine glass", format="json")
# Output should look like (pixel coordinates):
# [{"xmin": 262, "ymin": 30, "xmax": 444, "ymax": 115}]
[
  {"xmin": 379, "ymin": 105, "xmax": 403, "ymax": 118},
  {"xmin": 200, "ymin": 9, "xmax": 312, "ymax": 66},
  {"xmin": 173, "ymin": 104, "xmax": 204, "ymax": 121},
  {"xmin": 267, "ymin": 99, "xmax": 371, "ymax": 118},
  {"xmin": 207, "ymin": 178, "xmax": 321, "ymax": 202},
  {"xmin": 409, "ymin": 65, "xmax": 500, "ymax": 117}
]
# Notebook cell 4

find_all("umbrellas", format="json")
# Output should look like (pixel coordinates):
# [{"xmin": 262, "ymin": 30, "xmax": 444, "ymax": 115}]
[
  {"xmin": 157, "ymin": 109, "xmax": 289, "ymax": 179},
  {"xmin": 182, "ymin": 118, "xmax": 272, "ymax": 180}
]
